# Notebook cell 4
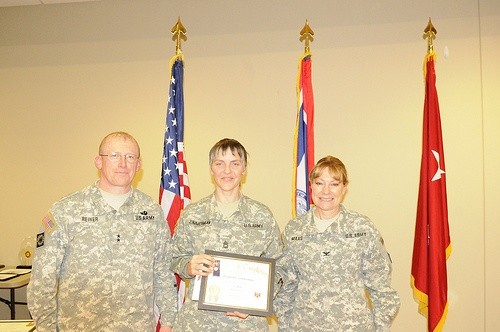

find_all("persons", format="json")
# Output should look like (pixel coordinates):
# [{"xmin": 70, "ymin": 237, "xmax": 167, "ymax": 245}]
[
  {"xmin": 26, "ymin": 132, "xmax": 178, "ymax": 332},
  {"xmin": 169, "ymin": 138, "xmax": 284, "ymax": 332},
  {"xmin": 272, "ymin": 155, "xmax": 400, "ymax": 332}
]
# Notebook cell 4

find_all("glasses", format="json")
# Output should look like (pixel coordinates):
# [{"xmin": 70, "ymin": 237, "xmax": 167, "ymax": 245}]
[{"xmin": 99, "ymin": 151, "xmax": 140, "ymax": 163}]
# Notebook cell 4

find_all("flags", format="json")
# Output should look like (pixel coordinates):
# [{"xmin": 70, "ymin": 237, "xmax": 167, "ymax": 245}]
[
  {"xmin": 290, "ymin": 20, "xmax": 315, "ymax": 220},
  {"xmin": 155, "ymin": 17, "xmax": 191, "ymax": 332},
  {"xmin": 411, "ymin": 17, "xmax": 453, "ymax": 332}
]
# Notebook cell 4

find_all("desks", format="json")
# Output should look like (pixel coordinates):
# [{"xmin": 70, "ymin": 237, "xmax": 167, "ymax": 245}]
[
  {"xmin": 0, "ymin": 320, "xmax": 36, "ymax": 332},
  {"xmin": 0, "ymin": 268, "xmax": 32, "ymax": 320}
]
